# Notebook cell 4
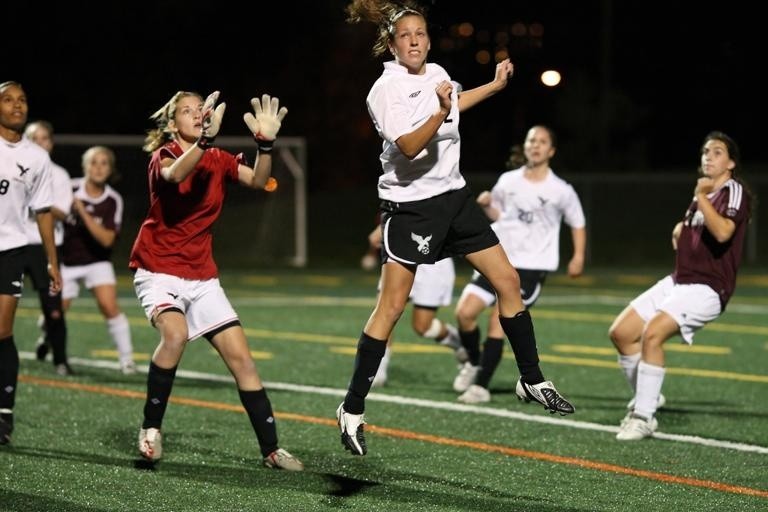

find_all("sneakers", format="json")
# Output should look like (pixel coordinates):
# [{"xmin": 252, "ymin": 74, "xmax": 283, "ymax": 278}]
[
  {"xmin": 35, "ymin": 334, "xmax": 138, "ymax": 375},
  {"xmin": 515, "ymin": 376, "xmax": 575, "ymax": 416},
  {"xmin": 261, "ymin": 447, "xmax": 303, "ymax": 473},
  {"xmin": 137, "ymin": 427, "xmax": 162, "ymax": 460},
  {"xmin": 1, "ymin": 406, "xmax": 15, "ymax": 443},
  {"xmin": 334, "ymin": 401, "xmax": 370, "ymax": 455},
  {"xmin": 616, "ymin": 389, "xmax": 666, "ymax": 441},
  {"xmin": 454, "ymin": 344, "xmax": 491, "ymax": 404}
]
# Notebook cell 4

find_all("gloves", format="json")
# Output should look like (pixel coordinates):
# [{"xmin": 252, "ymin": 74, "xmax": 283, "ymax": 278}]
[
  {"xmin": 243, "ymin": 93, "xmax": 288, "ymax": 154},
  {"xmin": 197, "ymin": 90, "xmax": 226, "ymax": 153}
]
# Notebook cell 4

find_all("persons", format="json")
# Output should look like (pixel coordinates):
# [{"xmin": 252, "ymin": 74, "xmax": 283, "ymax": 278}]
[
  {"xmin": 452, "ymin": 124, "xmax": 586, "ymax": 403},
  {"xmin": 0, "ymin": 81, "xmax": 136, "ymax": 446},
  {"xmin": 335, "ymin": 2, "xmax": 575, "ymax": 454},
  {"xmin": 364, "ymin": 220, "xmax": 469, "ymax": 385},
  {"xmin": 128, "ymin": 91, "xmax": 306, "ymax": 473},
  {"xmin": 609, "ymin": 132, "xmax": 751, "ymax": 442}
]
